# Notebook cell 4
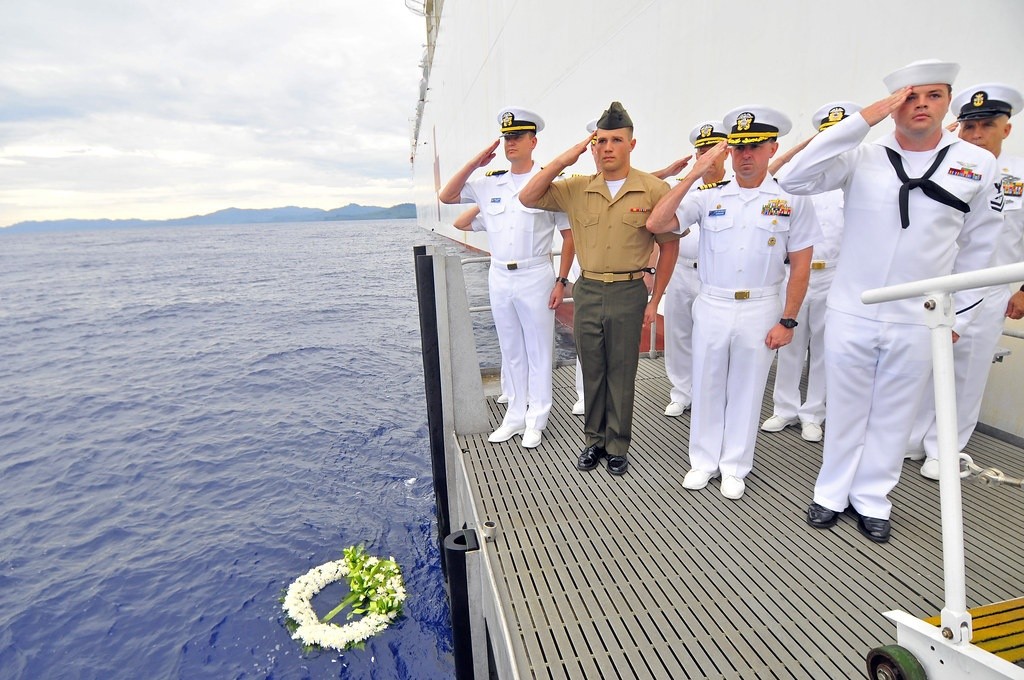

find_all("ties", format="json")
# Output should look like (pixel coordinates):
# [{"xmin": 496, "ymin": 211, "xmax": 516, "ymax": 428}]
[{"xmin": 886, "ymin": 144, "xmax": 970, "ymax": 230}]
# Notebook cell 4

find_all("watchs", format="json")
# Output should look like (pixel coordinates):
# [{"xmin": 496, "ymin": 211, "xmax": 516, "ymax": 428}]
[
  {"xmin": 556, "ymin": 277, "xmax": 569, "ymax": 287},
  {"xmin": 779, "ymin": 319, "xmax": 799, "ymax": 328},
  {"xmin": 642, "ymin": 267, "xmax": 656, "ymax": 274}
]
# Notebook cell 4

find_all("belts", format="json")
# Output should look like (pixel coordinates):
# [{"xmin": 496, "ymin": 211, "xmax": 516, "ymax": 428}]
[
  {"xmin": 491, "ymin": 254, "xmax": 551, "ymax": 271},
  {"xmin": 676, "ymin": 256, "xmax": 698, "ymax": 268},
  {"xmin": 579, "ymin": 270, "xmax": 645, "ymax": 283},
  {"xmin": 703, "ymin": 284, "xmax": 781, "ymax": 300},
  {"xmin": 809, "ymin": 260, "xmax": 836, "ymax": 269}
]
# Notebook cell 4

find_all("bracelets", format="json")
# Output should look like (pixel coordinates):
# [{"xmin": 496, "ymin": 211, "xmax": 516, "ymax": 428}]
[{"xmin": 1020, "ymin": 284, "xmax": 1024, "ymax": 292}]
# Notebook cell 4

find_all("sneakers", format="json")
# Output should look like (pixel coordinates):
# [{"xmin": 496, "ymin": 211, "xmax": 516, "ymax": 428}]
[
  {"xmin": 761, "ymin": 415, "xmax": 822, "ymax": 442},
  {"xmin": 904, "ymin": 453, "xmax": 939, "ymax": 480},
  {"xmin": 665, "ymin": 402, "xmax": 690, "ymax": 417},
  {"xmin": 681, "ymin": 469, "xmax": 745, "ymax": 499},
  {"xmin": 488, "ymin": 394, "xmax": 586, "ymax": 448}
]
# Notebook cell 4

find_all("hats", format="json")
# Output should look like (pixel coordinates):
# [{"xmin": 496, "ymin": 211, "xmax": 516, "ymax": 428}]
[
  {"xmin": 951, "ymin": 84, "xmax": 1024, "ymax": 121},
  {"xmin": 883, "ymin": 59, "xmax": 962, "ymax": 94},
  {"xmin": 722, "ymin": 105, "xmax": 793, "ymax": 146},
  {"xmin": 497, "ymin": 108, "xmax": 545, "ymax": 138},
  {"xmin": 811, "ymin": 101, "xmax": 863, "ymax": 133},
  {"xmin": 586, "ymin": 119, "xmax": 601, "ymax": 144},
  {"xmin": 689, "ymin": 121, "xmax": 730, "ymax": 149},
  {"xmin": 596, "ymin": 102, "xmax": 633, "ymax": 130}
]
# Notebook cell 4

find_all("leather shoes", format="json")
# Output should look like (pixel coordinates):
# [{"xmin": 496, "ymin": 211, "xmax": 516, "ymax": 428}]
[
  {"xmin": 807, "ymin": 501, "xmax": 839, "ymax": 528},
  {"xmin": 577, "ymin": 447, "xmax": 628, "ymax": 474},
  {"xmin": 858, "ymin": 514, "xmax": 891, "ymax": 542}
]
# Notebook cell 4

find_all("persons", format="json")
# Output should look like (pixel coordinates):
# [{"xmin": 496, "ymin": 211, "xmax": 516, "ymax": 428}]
[
  {"xmin": 778, "ymin": 61, "xmax": 1006, "ymax": 543},
  {"xmin": 904, "ymin": 85, "xmax": 1024, "ymax": 482},
  {"xmin": 648, "ymin": 122, "xmax": 728, "ymax": 418},
  {"xmin": 572, "ymin": 121, "xmax": 642, "ymax": 415},
  {"xmin": 762, "ymin": 103, "xmax": 863, "ymax": 441},
  {"xmin": 642, "ymin": 107, "xmax": 815, "ymax": 499},
  {"xmin": 438, "ymin": 108, "xmax": 556, "ymax": 448},
  {"xmin": 517, "ymin": 102, "xmax": 679, "ymax": 475},
  {"xmin": 452, "ymin": 206, "xmax": 512, "ymax": 403}
]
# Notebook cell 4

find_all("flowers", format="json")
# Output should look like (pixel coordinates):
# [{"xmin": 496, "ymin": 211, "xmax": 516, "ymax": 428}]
[{"xmin": 282, "ymin": 544, "xmax": 408, "ymax": 650}]
